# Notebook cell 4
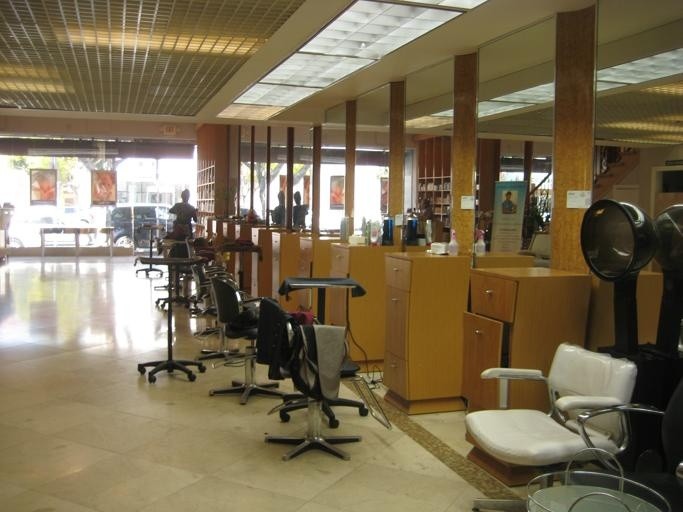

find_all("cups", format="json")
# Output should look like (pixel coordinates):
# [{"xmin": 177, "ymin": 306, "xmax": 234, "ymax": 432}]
[
  {"xmin": 406, "ymin": 219, "xmax": 417, "ymax": 246},
  {"xmin": 430, "ymin": 243, "xmax": 444, "ymax": 254},
  {"xmin": 433, "ymin": 185, "xmax": 441, "ymax": 191},
  {"xmin": 383, "ymin": 219, "xmax": 392, "ymax": 245},
  {"xmin": 416, "ymin": 238, "xmax": 425, "ymax": 246}
]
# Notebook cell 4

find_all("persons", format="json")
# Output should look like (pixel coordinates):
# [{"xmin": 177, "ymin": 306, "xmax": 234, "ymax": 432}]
[
  {"xmin": 421, "ymin": 198, "xmax": 433, "ymax": 221},
  {"xmin": 503, "ymin": 191, "xmax": 514, "ymax": 209},
  {"xmin": 293, "ymin": 191, "xmax": 307, "ymax": 230},
  {"xmin": 170, "ymin": 189, "xmax": 197, "ymax": 240},
  {"xmin": 271, "ymin": 191, "xmax": 285, "ymax": 226}
]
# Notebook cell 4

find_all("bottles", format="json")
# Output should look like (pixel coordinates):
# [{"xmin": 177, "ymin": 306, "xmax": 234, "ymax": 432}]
[
  {"xmin": 425, "ymin": 220, "xmax": 431, "ymax": 244},
  {"xmin": 362, "ymin": 216, "xmax": 382, "ymax": 245},
  {"xmin": 339, "ymin": 217, "xmax": 349, "ymax": 240}
]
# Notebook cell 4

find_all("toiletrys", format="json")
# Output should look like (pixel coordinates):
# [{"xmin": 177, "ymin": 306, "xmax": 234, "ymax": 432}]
[
  {"xmin": 425, "ymin": 219, "xmax": 433, "ymax": 247},
  {"xmin": 370, "ymin": 223, "xmax": 379, "ymax": 248}
]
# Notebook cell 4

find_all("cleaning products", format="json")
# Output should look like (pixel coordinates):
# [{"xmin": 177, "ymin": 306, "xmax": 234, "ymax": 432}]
[
  {"xmin": 474, "ymin": 228, "xmax": 486, "ymax": 257},
  {"xmin": 448, "ymin": 229, "xmax": 459, "ymax": 256}
]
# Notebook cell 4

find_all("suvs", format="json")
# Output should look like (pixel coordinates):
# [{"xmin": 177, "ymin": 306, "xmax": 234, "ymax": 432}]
[{"xmin": 105, "ymin": 204, "xmax": 195, "ymax": 252}]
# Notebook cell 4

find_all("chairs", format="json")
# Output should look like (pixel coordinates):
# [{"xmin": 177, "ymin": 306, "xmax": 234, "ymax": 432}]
[
  {"xmin": 458, "ymin": 339, "xmax": 640, "ymax": 511},
  {"xmin": 255, "ymin": 297, "xmax": 364, "ymax": 461},
  {"xmin": 571, "ymin": 375, "xmax": 683, "ymax": 511},
  {"xmin": 211, "ymin": 280, "xmax": 286, "ymax": 411},
  {"xmin": 130, "ymin": 218, "xmax": 246, "ymax": 384}
]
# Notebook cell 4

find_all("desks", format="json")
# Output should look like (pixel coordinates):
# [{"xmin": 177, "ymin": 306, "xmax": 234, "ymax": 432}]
[
  {"xmin": 38, "ymin": 227, "xmax": 96, "ymax": 260},
  {"xmin": 279, "ymin": 276, "xmax": 368, "ymax": 430}
]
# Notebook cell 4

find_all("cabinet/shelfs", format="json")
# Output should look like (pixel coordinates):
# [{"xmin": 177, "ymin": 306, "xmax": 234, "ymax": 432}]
[
  {"xmin": 379, "ymin": 250, "xmax": 470, "ymax": 417},
  {"xmin": 195, "ymin": 159, "xmax": 216, "ymax": 214},
  {"xmin": 458, "ymin": 266, "xmax": 595, "ymax": 485},
  {"xmin": 323, "ymin": 241, "xmax": 400, "ymax": 376}
]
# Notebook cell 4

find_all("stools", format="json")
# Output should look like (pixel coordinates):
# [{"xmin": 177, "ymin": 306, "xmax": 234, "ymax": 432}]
[{"xmin": 98, "ymin": 228, "xmax": 118, "ymax": 261}]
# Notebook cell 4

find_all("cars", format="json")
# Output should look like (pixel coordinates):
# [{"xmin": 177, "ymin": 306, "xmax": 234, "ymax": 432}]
[
  {"xmin": 3, "ymin": 211, "xmax": 98, "ymax": 247},
  {"xmin": 233, "ymin": 208, "xmax": 261, "ymax": 222}
]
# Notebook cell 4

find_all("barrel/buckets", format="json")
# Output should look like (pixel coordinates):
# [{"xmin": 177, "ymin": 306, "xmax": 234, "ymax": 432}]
[{"xmin": 526, "ymin": 448, "xmax": 673, "ymax": 512}]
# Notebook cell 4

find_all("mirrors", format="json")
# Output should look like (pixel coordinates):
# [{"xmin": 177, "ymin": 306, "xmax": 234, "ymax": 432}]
[{"xmin": 222, "ymin": 1, "xmax": 683, "ymax": 275}]
